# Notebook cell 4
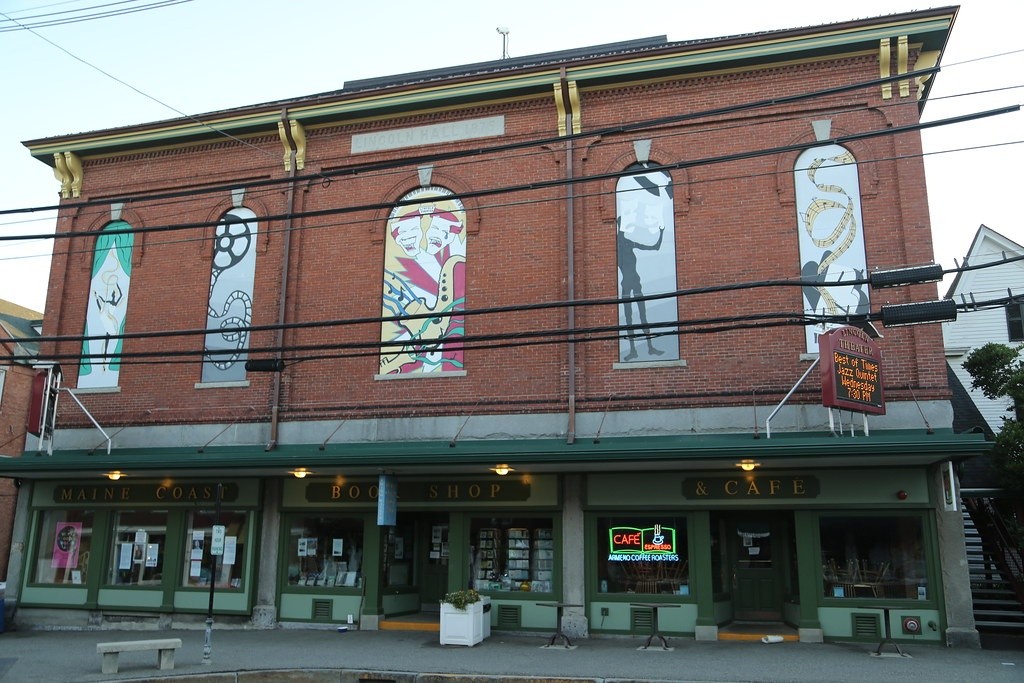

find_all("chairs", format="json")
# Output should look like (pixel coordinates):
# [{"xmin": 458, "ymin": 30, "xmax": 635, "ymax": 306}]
[
  {"xmin": 862, "ymin": 559, "xmax": 890, "ymax": 599},
  {"xmin": 632, "ymin": 561, "xmax": 662, "ymax": 594},
  {"xmin": 827, "ymin": 559, "xmax": 859, "ymax": 598},
  {"xmin": 664, "ymin": 560, "xmax": 688, "ymax": 595}
]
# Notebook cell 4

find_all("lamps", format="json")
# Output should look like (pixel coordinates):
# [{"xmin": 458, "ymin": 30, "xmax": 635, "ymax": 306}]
[
  {"xmin": 735, "ymin": 459, "xmax": 760, "ymax": 471},
  {"xmin": 488, "ymin": 463, "xmax": 516, "ymax": 476},
  {"xmin": 287, "ymin": 467, "xmax": 315, "ymax": 478},
  {"xmin": 101, "ymin": 469, "xmax": 128, "ymax": 481}
]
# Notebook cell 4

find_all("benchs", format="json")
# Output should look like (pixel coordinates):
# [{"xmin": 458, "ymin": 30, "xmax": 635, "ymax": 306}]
[{"xmin": 96, "ymin": 638, "xmax": 182, "ymax": 674}]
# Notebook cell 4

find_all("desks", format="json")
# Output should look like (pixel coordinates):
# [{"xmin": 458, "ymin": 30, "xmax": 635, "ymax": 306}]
[
  {"xmin": 536, "ymin": 601, "xmax": 584, "ymax": 649},
  {"xmin": 630, "ymin": 603, "xmax": 681, "ymax": 650},
  {"xmin": 623, "ymin": 578, "xmax": 678, "ymax": 586},
  {"xmin": 857, "ymin": 605, "xmax": 912, "ymax": 658},
  {"xmin": 835, "ymin": 584, "xmax": 871, "ymax": 588}
]
